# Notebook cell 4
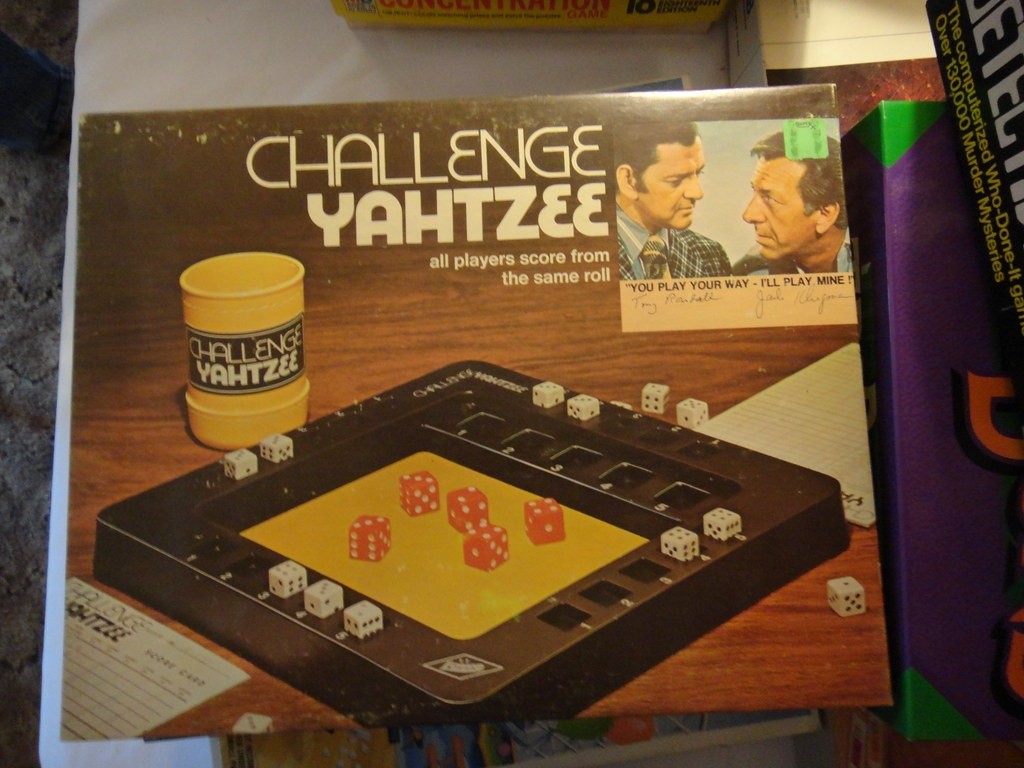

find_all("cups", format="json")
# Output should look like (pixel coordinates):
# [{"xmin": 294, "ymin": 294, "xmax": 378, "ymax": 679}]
[{"xmin": 177, "ymin": 250, "xmax": 309, "ymax": 451}]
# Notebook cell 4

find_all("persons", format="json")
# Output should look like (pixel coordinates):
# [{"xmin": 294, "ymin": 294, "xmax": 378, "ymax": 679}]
[
  {"xmin": 614, "ymin": 113, "xmax": 736, "ymax": 278},
  {"xmin": 732, "ymin": 130, "xmax": 853, "ymax": 275}
]
